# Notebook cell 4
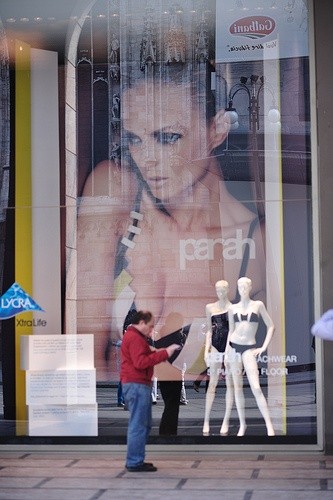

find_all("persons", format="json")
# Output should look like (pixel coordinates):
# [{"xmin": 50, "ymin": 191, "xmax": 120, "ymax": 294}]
[
  {"xmin": 115, "ymin": 330, "xmax": 124, "ymax": 406},
  {"xmin": 120, "ymin": 309, "xmax": 179, "ymax": 472},
  {"xmin": 147, "ymin": 376, "xmax": 159, "ymax": 406},
  {"xmin": 180, "ymin": 363, "xmax": 190, "ymax": 405},
  {"xmin": 76, "ymin": 51, "xmax": 265, "ymax": 381},
  {"xmin": 225, "ymin": 277, "xmax": 275, "ymax": 436},
  {"xmin": 147, "ymin": 326, "xmax": 159, "ymax": 350},
  {"xmin": 203, "ymin": 280, "xmax": 233, "ymax": 433},
  {"xmin": 193, "ymin": 326, "xmax": 216, "ymax": 394},
  {"xmin": 159, "ymin": 312, "xmax": 183, "ymax": 435}
]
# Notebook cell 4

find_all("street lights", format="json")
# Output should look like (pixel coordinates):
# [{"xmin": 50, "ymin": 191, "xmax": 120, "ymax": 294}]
[{"xmin": 224, "ymin": 80, "xmax": 281, "ymax": 225}]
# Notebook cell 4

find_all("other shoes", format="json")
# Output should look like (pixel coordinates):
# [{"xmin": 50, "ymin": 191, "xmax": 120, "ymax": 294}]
[{"xmin": 126, "ymin": 461, "xmax": 156, "ymax": 472}]
